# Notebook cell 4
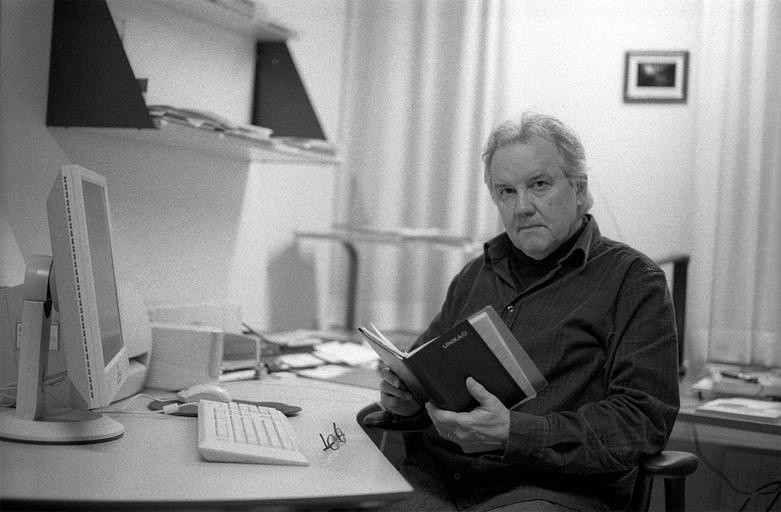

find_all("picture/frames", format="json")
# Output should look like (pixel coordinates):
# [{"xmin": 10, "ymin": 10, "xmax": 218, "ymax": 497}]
[{"xmin": 621, "ymin": 47, "xmax": 691, "ymax": 104}]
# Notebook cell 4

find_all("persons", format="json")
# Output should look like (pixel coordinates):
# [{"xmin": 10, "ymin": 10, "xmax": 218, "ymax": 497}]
[{"xmin": 356, "ymin": 109, "xmax": 683, "ymax": 511}]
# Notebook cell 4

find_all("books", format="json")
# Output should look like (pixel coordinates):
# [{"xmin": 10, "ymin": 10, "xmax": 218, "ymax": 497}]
[
  {"xmin": 696, "ymin": 395, "xmax": 781, "ymax": 424},
  {"xmin": 355, "ymin": 305, "xmax": 552, "ymax": 412}
]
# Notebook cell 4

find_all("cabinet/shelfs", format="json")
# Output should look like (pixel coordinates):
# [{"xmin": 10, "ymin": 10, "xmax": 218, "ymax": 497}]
[{"xmin": 46, "ymin": 0, "xmax": 343, "ymax": 168}]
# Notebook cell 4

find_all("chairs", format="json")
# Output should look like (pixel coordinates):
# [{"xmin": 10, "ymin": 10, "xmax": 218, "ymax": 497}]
[{"xmin": 359, "ymin": 255, "xmax": 701, "ymax": 507}]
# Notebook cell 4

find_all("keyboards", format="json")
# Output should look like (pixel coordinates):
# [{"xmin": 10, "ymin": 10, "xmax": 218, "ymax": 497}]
[{"xmin": 197, "ymin": 398, "xmax": 310, "ymax": 466}]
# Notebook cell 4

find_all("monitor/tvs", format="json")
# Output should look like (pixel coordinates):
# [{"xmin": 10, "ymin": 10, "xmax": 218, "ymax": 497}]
[{"xmin": 0, "ymin": 162, "xmax": 132, "ymax": 444}]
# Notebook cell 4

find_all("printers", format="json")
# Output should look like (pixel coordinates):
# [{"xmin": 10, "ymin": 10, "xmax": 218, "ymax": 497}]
[{"xmin": 0, "ymin": 277, "xmax": 153, "ymax": 409}]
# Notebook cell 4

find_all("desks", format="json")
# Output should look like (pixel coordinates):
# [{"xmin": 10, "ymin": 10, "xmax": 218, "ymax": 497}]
[
  {"xmin": 0, "ymin": 329, "xmax": 416, "ymax": 509},
  {"xmin": 654, "ymin": 369, "xmax": 781, "ymax": 509}
]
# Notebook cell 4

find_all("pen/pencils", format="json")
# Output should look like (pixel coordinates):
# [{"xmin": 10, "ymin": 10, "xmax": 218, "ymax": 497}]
[{"xmin": 721, "ymin": 370, "xmax": 759, "ymax": 383}]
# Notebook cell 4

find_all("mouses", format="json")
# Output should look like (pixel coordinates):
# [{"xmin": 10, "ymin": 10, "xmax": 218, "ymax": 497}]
[{"xmin": 176, "ymin": 382, "xmax": 232, "ymax": 403}]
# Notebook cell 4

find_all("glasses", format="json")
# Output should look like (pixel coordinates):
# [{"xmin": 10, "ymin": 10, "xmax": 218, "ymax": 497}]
[{"xmin": 320, "ymin": 422, "xmax": 346, "ymax": 451}]
